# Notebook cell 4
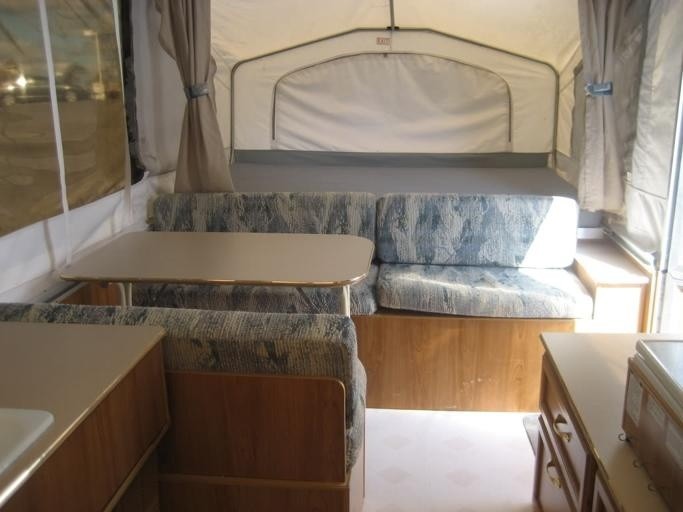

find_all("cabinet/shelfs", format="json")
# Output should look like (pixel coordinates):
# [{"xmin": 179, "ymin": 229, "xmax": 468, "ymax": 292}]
[
  {"xmin": 591, "ymin": 471, "xmax": 617, "ymax": 512},
  {"xmin": 532, "ymin": 352, "xmax": 594, "ymax": 512}
]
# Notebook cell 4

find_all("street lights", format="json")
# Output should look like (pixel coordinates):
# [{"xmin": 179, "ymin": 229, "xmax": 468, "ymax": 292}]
[{"xmin": 83, "ymin": 28, "xmax": 106, "ymax": 101}]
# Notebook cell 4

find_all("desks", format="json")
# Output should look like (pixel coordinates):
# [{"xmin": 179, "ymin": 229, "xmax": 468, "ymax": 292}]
[{"xmin": 59, "ymin": 231, "xmax": 375, "ymax": 318}]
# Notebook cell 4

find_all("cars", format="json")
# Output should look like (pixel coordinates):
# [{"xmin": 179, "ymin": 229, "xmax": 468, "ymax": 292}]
[{"xmin": 0, "ymin": 74, "xmax": 87, "ymax": 105}]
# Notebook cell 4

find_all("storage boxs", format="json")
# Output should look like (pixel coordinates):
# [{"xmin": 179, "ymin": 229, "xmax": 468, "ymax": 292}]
[{"xmin": 618, "ymin": 337, "xmax": 683, "ymax": 512}]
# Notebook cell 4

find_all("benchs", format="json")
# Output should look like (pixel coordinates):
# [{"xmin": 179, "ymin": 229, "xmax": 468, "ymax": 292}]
[{"xmin": 0, "ymin": 301, "xmax": 367, "ymax": 511}]
